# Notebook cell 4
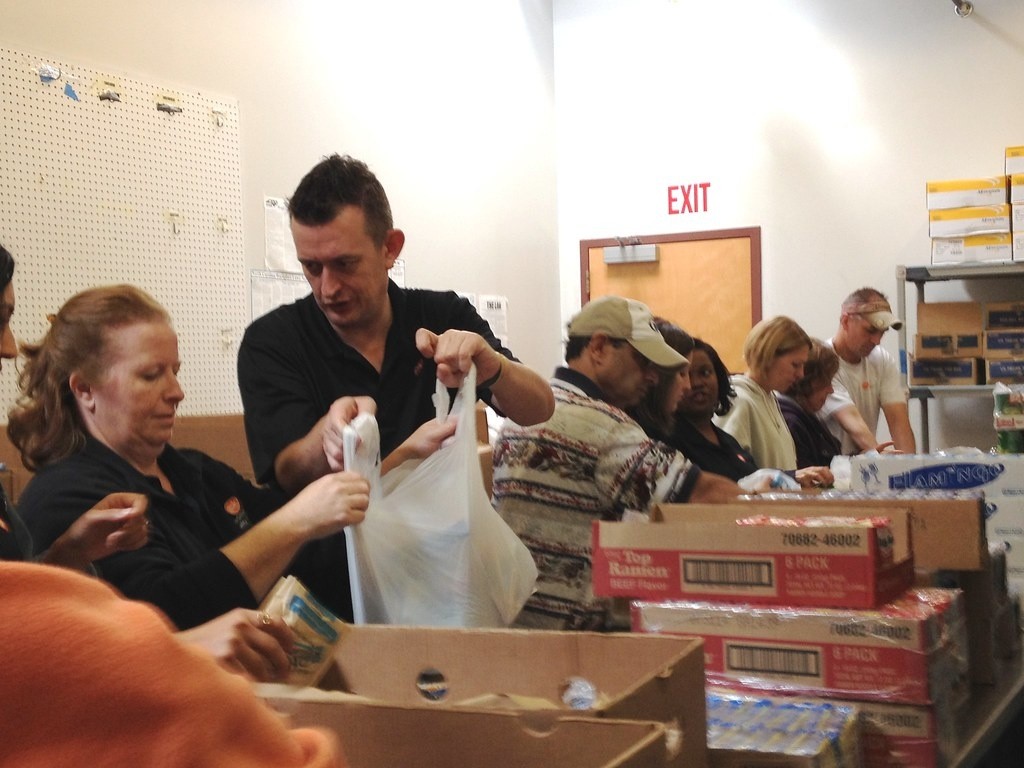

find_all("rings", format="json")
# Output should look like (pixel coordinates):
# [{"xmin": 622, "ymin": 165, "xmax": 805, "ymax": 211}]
[{"xmin": 257, "ymin": 612, "xmax": 270, "ymax": 623}]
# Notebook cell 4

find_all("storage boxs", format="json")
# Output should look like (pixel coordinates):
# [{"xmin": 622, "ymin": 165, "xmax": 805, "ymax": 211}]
[
  {"xmin": 909, "ymin": 303, "xmax": 1024, "ymax": 386},
  {"xmin": 259, "ymin": 493, "xmax": 1024, "ymax": 768},
  {"xmin": 927, "ymin": 144, "xmax": 1024, "ymax": 264}
]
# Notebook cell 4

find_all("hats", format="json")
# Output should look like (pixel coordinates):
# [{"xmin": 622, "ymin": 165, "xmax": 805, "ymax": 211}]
[
  {"xmin": 568, "ymin": 295, "xmax": 690, "ymax": 375},
  {"xmin": 842, "ymin": 302, "xmax": 902, "ymax": 332}
]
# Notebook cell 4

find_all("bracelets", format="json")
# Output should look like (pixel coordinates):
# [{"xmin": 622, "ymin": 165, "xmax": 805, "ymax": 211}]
[{"xmin": 479, "ymin": 364, "xmax": 502, "ymax": 388}]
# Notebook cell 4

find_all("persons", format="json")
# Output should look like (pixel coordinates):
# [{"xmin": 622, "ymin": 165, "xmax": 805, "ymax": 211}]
[
  {"xmin": 238, "ymin": 154, "xmax": 555, "ymax": 496},
  {"xmin": 0, "ymin": 242, "xmax": 455, "ymax": 768},
  {"xmin": 813, "ymin": 287, "xmax": 915, "ymax": 455},
  {"xmin": 486, "ymin": 294, "xmax": 821, "ymax": 632},
  {"xmin": 711, "ymin": 316, "xmax": 813, "ymax": 472},
  {"xmin": 629, "ymin": 318, "xmax": 834, "ymax": 488},
  {"xmin": 776, "ymin": 338, "xmax": 842, "ymax": 467}
]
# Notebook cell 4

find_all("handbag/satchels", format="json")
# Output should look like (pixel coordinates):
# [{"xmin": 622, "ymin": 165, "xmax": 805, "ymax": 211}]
[{"xmin": 340, "ymin": 361, "xmax": 540, "ymax": 628}]
